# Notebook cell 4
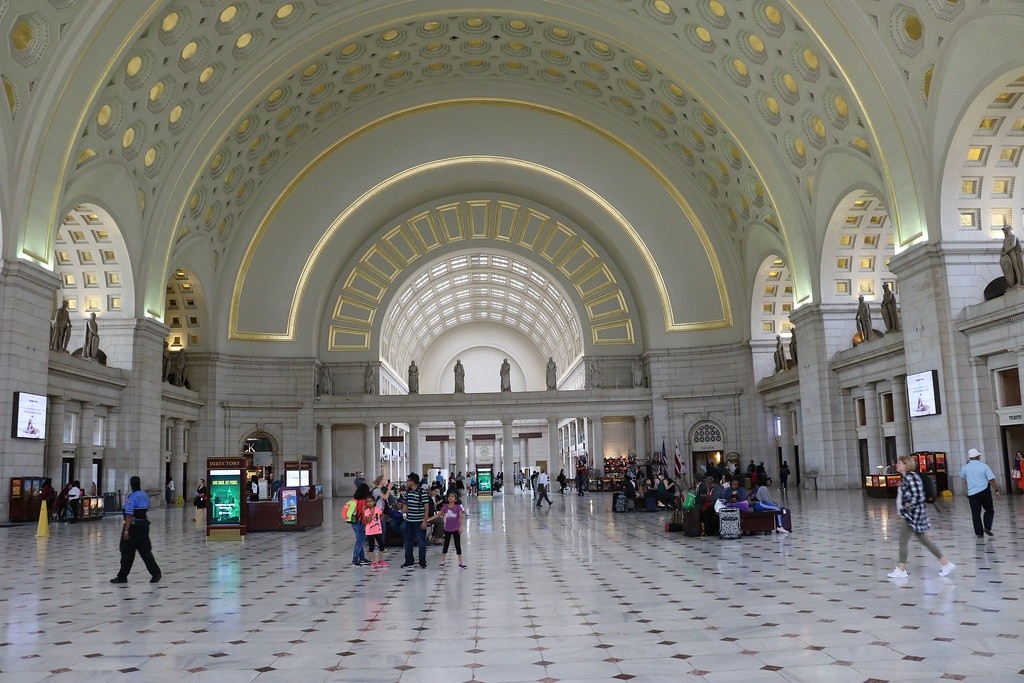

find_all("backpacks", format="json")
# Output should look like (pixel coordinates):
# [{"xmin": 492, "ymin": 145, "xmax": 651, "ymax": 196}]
[
  {"xmin": 747, "ymin": 486, "xmax": 764, "ymax": 506},
  {"xmin": 341, "ymin": 499, "xmax": 362, "ymax": 524},
  {"xmin": 911, "ymin": 471, "xmax": 935, "ymax": 504},
  {"xmin": 419, "ymin": 488, "xmax": 434, "ymax": 527}
]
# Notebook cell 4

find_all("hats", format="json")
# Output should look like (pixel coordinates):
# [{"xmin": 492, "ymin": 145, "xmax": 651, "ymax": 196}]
[{"xmin": 967, "ymin": 449, "xmax": 981, "ymax": 458}]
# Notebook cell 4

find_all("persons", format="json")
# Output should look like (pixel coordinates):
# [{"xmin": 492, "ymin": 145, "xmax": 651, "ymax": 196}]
[
  {"xmin": 855, "ymin": 295, "xmax": 872, "ymax": 339},
  {"xmin": 365, "ymin": 361, "xmax": 374, "ymax": 393},
  {"xmin": 408, "ymin": 360, "xmax": 419, "ymax": 392},
  {"xmin": 42, "ymin": 460, "xmax": 790, "ymax": 583},
  {"xmin": 546, "ymin": 357, "xmax": 556, "ymax": 388},
  {"xmin": 454, "ymin": 360, "xmax": 464, "ymax": 391},
  {"xmin": 886, "ymin": 455, "xmax": 956, "ymax": 577},
  {"xmin": 86, "ymin": 313, "xmax": 98, "ymax": 359},
  {"xmin": 959, "ymin": 448, "xmax": 1001, "ymax": 537},
  {"xmin": 1014, "ymin": 451, "xmax": 1024, "ymax": 492},
  {"xmin": 1001, "ymin": 225, "xmax": 1021, "ymax": 288},
  {"xmin": 776, "ymin": 328, "xmax": 796, "ymax": 370},
  {"xmin": 589, "ymin": 357, "xmax": 601, "ymax": 387},
  {"xmin": 632, "ymin": 356, "xmax": 644, "ymax": 386},
  {"xmin": 501, "ymin": 359, "xmax": 510, "ymax": 390},
  {"xmin": 163, "ymin": 341, "xmax": 186, "ymax": 386},
  {"xmin": 880, "ymin": 283, "xmax": 895, "ymax": 329},
  {"xmin": 55, "ymin": 300, "xmax": 72, "ymax": 352},
  {"xmin": 322, "ymin": 362, "xmax": 332, "ymax": 393}
]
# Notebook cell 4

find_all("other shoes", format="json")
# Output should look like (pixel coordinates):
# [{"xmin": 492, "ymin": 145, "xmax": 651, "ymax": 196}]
[
  {"xmin": 459, "ymin": 565, "xmax": 467, "ymax": 568},
  {"xmin": 536, "ymin": 504, "xmax": 543, "ymax": 507},
  {"xmin": 150, "ymin": 574, "xmax": 161, "ymax": 583},
  {"xmin": 433, "ymin": 543, "xmax": 444, "ymax": 546},
  {"xmin": 777, "ymin": 527, "xmax": 788, "ymax": 533},
  {"xmin": 780, "ymin": 488, "xmax": 792, "ymax": 533},
  {"xmin": 439, "ymin": 564, "xmax": 444, "ymax": 567},
  {"xmin": 976, "ymin": 528, "xmax": 993, "ymax": 538},
  {"xmin": 658, "ymin": 503, "xmax": 665, "ymax": 506},
  {"xmin": 549, "ymin": 501, "xmax": 553, "ymax": 505},
  {"xmin": 110, "ymin": 578, "xmax": 127, "ymax": 583}
]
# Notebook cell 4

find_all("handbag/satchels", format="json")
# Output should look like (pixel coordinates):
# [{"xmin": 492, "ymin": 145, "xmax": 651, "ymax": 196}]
[
  {"xmin": 1012, "ymin": 469, "xmax": 1021, "ymax": 478},
  {"xmin": 681, "ymin": 492, "xmax": 696, "ymax": 510},
  {"xmin": 714, "ymin": 499, "xmax": 727, "ymax": 513}
]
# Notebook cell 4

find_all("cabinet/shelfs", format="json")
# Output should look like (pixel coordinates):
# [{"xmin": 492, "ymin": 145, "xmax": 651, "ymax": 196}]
[{"xmin": 7, "ymin": 476, "xmax": 53, "ymax": 525}]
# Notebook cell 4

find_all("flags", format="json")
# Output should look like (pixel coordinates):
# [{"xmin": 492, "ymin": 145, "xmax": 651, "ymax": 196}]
[
  {"xmin": 674, "ymin": 445, "xmax": 682, "ymax": 477},
  {"xmin": 663, "ymin": 441, "xmax": 667, "ymax": 473}
]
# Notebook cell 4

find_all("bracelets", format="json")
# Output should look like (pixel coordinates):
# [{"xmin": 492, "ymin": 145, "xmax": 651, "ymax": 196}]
[
  {"xmin": 124, "ymin": 532, "xmax": 129, "ymax": 534},
  {"xmin": 994, "ymin": 489, "xmax": 999, "ymax": 492}
]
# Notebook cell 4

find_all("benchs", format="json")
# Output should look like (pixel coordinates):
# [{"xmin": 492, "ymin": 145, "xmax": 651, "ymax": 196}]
[
  {"xmin": 696, "ymin": 509, "xmax": 782, "ymax": 538},
  {"xmin": 384, "ymin": 523, "xmax": 434, "ymax": 549}
]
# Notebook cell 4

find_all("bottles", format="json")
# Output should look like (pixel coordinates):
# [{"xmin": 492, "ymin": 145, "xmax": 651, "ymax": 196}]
[{"xmin": 315, "ymin": 481, "xmax": 323, "ymax": 498}]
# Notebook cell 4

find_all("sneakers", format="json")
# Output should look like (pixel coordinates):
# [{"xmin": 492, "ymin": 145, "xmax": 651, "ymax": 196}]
[
  {"xmin": 401, "ymin": 563, "xmax": 427, "ymax": 570},
  {"xmin": 887, "ymin": 568, "xmax": 908, "ymax": 577},
  {"xmin": 939, "ymin": 561, "xmax": 956, "ymax": 576},
  {"xmin": 351, "ymin": 560, "xmax": 389, "ymax": 568}
]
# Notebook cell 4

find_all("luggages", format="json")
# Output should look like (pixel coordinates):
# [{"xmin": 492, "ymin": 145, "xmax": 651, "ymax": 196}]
[
  {"xmin": 719, "ymin": 496, "xmax": 743, "ymax": 539},
  {"xmin": 683, "ymin": 507, "xmax": 702, "ymax": 538},
  {"xmin": 616, "ymin": 498, "xmax": 628, "ymax": 513}
]
